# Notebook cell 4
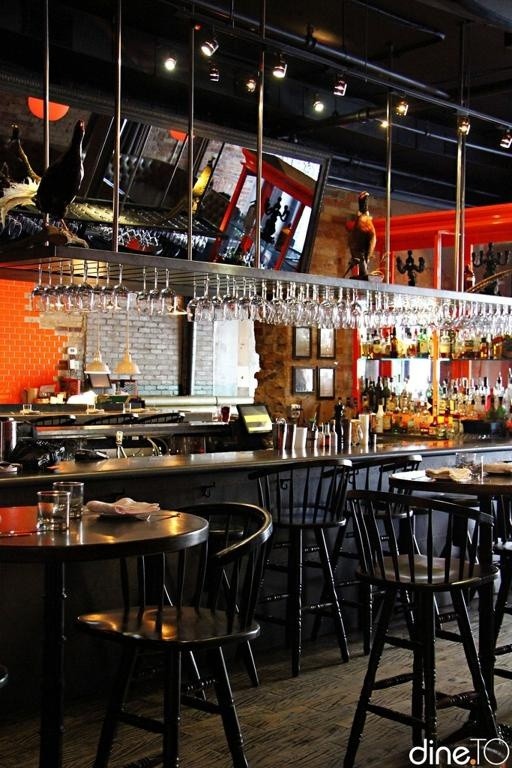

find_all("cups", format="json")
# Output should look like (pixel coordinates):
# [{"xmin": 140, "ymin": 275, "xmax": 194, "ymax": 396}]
[
  {"xmin": 342, "ymin": 413, "xmax": 370, "ymax": 445},
  {"xmin": 454, "ymin": 451, "xmax": 485, "ymax": 483},
  {"xmin": 37, "ymin": 490, "xmax": 70, "ymax": 531},
  {"xmin": 221, "ymin": 403, "xmax": 231, "ymax": 422},
  {"xmin": 122, "ymin": 403, "xmax": 132, "ymax": 413},
  {"xmin": 212, "ymin": 410, "xmax": 219, "ymax": 421},
  {"xmin": 86, "ymin": 403, "xmax": 96, "ymax": 413},
  {"xmin": 1, "ymin": 421, "xmax": 17, "ymax": 458},
  {"xmin": 22, "ymin": 403, "xmax": 33, "ymax": 414},
  {"xmin": 273, "ymin": 423, "xmax": 307, "ymax": 450},
  {"xmin": 52, "ymin": 481, "xmax": 84, "ymax": 519}
]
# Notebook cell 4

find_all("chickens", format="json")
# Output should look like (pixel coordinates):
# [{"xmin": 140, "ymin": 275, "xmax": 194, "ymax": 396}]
[
  {"xmin": 0, "ymin": 119, "xmax": 89, "ymax": 249},
  {"xmin": 0, "ymin": 125, "xmax": 42, "ymax": 188}
]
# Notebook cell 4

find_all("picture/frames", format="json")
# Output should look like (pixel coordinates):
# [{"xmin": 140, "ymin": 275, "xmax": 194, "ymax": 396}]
[
  {"xmin": 317, "ymin": 366, "xmax": 336, "ymax": 401},
  {"xmin": 315, "ymin": 328, "xmax": 337, "ymax": 361},
  {"xmin": 291, "ymin": 365, "xmax": 316, "ymax": 394},
  {"xmin": 290, "ymin": 325, "xmax": 313, "ymax": 360}
]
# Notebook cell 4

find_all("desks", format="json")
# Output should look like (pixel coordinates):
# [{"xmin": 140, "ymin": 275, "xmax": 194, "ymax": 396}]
[
  {"xmin": 2, "ymin": 503, "xmax": 211, "ymax": 767},
  {"xmin": 383, "ymin": 464, "xmax": 510, "ymax": 760}
]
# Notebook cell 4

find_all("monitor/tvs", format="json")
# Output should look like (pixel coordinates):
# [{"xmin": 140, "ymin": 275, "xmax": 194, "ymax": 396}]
[{"xmin": 236, "ymin": 403, "xmax": 274, "ymax": 434}]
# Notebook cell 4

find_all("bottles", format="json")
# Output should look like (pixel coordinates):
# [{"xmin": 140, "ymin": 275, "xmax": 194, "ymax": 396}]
[
  {"xmin": 307, "ymin": 419, "xmax": 337, "ymax": 448},
  {"xmin": 362, "ymin": 367, "xmax": 512, "ymax": 437},
  {"xmin": 335, "ymin": 397, "xmax": 358, "ymax": 418}
]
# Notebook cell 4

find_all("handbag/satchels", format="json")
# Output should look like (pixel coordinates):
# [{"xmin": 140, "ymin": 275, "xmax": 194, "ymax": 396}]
[{"xmin": 11, "ymin": 438, "xmax": 66, "ymax": 468}]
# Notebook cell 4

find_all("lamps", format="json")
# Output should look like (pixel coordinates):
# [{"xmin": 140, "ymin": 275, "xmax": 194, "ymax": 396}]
[
  {"xmin": 112, "ymin": 312, "xmax": 144, "ymax": 376},
  {"xmin": 83, "ymin": 308, "xmax": 112, "ymax": 375},
  {"xmin": 159, "ymin": 26, "xmax": 512, "ymax": 168}
]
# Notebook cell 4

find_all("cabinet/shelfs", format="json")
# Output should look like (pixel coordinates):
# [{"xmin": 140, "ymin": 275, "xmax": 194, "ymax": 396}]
[{"xmin": 354, "ymin": 305, "xmax": 512, "ymax": 440}]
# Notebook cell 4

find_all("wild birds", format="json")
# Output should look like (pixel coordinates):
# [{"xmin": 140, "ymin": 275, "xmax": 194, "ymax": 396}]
[
  {"xmin": 343, "ymin": 190, "xmax": 383, "ymax": 279},
  {"xmin": 155, "ymin": 156, "xmax": 216, "ymax": 224}
]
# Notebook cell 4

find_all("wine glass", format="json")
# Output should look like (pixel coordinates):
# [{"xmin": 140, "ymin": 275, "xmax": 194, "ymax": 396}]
[{"xmin": 29, "ymin": 261, "xmax": 510, "ymax": 338}]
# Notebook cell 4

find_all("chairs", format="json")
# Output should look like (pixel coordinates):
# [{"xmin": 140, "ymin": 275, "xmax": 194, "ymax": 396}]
[
  {"xmin": 239, "ymin": 456, "xmax": 356, "ymax": 680},
  {"xmin": 340, "ymin": 487, "xmax": 511, "ymax": 767},
  {"xmin": 91, "ymin": 498, "xmax": 276, "ymax": 767},
  {"xmin": 304, "ymin": 452, "xmax": 428, "ymax": 659}
]
